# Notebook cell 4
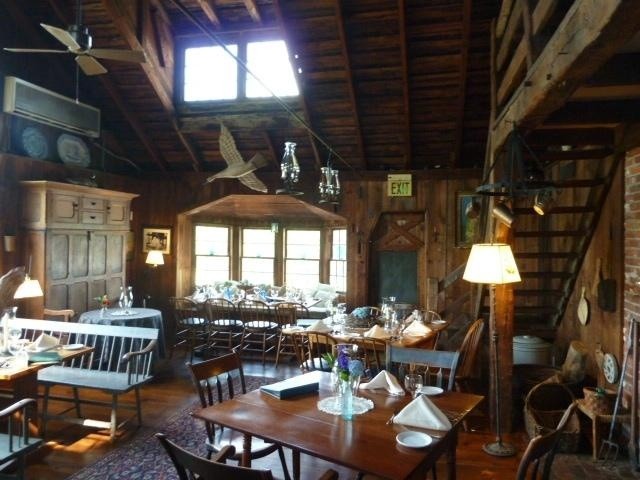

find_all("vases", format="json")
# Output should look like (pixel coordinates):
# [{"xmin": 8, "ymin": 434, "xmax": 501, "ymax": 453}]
[
  {"xmin": 337, "ymin": 383, "xmax": 355, "ymax": 422},
  {"xmin": 100, "ymin": 307, "xmax": 107, "ymax": 318}
]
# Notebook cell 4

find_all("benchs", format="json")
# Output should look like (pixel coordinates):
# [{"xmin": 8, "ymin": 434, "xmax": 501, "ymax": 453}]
[
  {"xmin": 0, "ymin": 397, "xmax": 45, "ymax": 480},
  {"xmin": 15, "ymin": 318, "xmax": 159, "ymax": 443}
]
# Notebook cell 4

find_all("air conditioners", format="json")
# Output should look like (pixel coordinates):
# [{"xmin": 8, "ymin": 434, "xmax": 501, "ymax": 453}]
[{"xmin": 3, "ymin": 76, "xmax": 102, "ymax": 138}]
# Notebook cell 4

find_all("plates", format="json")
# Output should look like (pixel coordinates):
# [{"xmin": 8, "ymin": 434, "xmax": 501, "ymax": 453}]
[
  {"xmin": 432, "ymin": 320, "xmax": 447, "ymax": 325},
  {"xmin": 396, "ymin": 431, "xmax": 433, "ymax": 448},
  {"xmin": 420, "ymin": 386, "xmax": 444, "ymax": 395},
  {"xmin": 347, "ymin": 333, "xmax": 361, "ymax": 337},
  {"xmin": 62, "ymin": 344, "xmax": 83, "ymax": 350}
]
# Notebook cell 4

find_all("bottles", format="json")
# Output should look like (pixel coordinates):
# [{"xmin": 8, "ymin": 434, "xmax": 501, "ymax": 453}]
[
  {"xmin": 101, "ymin": 307, "xmax": 107, "ymax": 318},
  {"xmin": 228, "ymin": 283, "xmax": 233, "ymax": 298},
  {"xmin": 341, "ymin": 378, "xmax": 353, "ymax": 421},
  {"xmin": 261, "ymin": 289, "xmax": 265, "ymax": 299}
]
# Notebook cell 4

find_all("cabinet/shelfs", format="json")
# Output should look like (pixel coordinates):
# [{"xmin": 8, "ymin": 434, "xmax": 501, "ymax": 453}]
[{"xmin": 16, "ymin": 180, "xmax": 140, "ymax": 322}]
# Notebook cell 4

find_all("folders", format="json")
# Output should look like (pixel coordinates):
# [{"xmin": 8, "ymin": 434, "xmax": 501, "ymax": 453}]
[{"xmin": 260, "ymin": 376, "xmax": 319, "ymax": 400}]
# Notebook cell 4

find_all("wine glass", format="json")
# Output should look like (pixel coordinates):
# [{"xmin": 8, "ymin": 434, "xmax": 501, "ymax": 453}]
[
  {"xmin": 254, "ymin": 287, "xmax": 260, "ymax": 300},
  {"xmin": 404, "ymin": 374, "xmax": 424, "ymax": 400},
  {"xmin": 328, "ymin": 299, "xmax": 337, "ymax": 325}
]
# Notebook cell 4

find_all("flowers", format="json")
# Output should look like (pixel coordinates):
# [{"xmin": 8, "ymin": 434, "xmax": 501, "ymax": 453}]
[
  {"xmin": 321, "ymin": 344, "xmax": 366, "ymax": 388},
  {"xmin": 94, "ymin": 295, "xmax": 113, "ymax": 307}
]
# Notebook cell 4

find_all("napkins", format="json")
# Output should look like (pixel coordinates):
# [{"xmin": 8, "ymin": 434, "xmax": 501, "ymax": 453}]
[
  {"xmin": 360, "ymin": 369, "xmax": 406, "ymax": 397},
  {"xmin": 362, "ymin": 323, "xmax": 391, "ymax": 341},
  {"xmin": 402, "ymin": 307, "xmax": 442, "ymax": 326},
  {"xmin": 405, "ymin": 310, "xmax": 423, "ymax": 322},
  {"xmin": 392, "ymin": 392, "xmax": 453, "ymax": 432},
  {"xmin": 402, "ymin": 319, "xmax": 432, "ymax": 339}
]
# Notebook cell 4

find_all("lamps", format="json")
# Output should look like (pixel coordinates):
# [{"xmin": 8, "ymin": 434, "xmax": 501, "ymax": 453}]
[
  {"xmin": 145, "ymin": 249, "xmax": 164, "ymax": 266},
  {"xmin": 463, "ymin": 243, "xmax": 522, "ymax": 458},
  {"xmin": 276, "ymin": 113, "xmax": 341, "ymax": 204},
  {"xmin": 466, "ymin": 122, "xmax": 552, "ymax": 228},
  {"xmin": 14, "ymin": 274, "xmax": 44, "ymax": 299}
]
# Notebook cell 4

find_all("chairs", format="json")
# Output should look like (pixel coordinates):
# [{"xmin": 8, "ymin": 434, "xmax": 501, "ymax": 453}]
[
  {"xmin": 156, "ymin": 429, "xmax": 344, "ymax": 479},
  {"xmin": 356, "ymin": 305, "xmax": 385, "ymax": 320},
  {"xmin": 345, "ymin": 334, "xmax": 396, "ymax": 388},
  {"xmin": 290, "ymin": 330, "xmax": 341, "ymax": 373},
  {"xmin": 190, "ymin": 349, "xmax": 292, "ymax": 479},
  {"xmin": 44, "ymin": 308, "xmax": 74, "ymax": 344},
  {"xmin": 273, "ymin": 301, "xmax": 314, "ymax": 366},
  {"xmin": 202, "ymin": 297, "xmax": 242, "ymax": 361},
  {"xmin": 237, "ymin": 298, "xmax": 280, "ymax": 368},
  {"xmin": 427, "ymin": 317, "xmax": 482, "ymax": 394},
  {"xmin": 165, "ymin": 298, "xmax": 210, "ymax": 358},
  {"xmin": 383, "ymin": 344, "xmax": 461, "ymax": 480},
  {"xmin": 512, "ymin": 428, "xmax": 565, "ymax": 478}
]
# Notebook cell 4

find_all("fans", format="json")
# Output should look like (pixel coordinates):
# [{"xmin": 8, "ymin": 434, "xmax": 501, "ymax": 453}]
[{"xmin": 3, "ymin": 0, "xmax": 146, "ymax": 75}]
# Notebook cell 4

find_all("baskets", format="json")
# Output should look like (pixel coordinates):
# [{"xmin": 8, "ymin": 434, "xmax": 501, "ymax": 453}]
[
  {"xmin": 583, "ymin": 387, "xmax": 617, "ymax": 413},
  {"xmin": 524, "ymin": 381, "xmax": 581, "ymax": 453}
]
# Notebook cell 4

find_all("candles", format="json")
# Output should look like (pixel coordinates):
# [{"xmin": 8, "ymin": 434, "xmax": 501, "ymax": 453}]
[{"xmin": 125, "ymin": 296, "xmax": 128, "ymax": 305}]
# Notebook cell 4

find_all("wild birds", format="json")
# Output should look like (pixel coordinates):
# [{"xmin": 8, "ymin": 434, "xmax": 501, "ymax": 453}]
[{"xmin": 201, "ymin": 123, "xmax": 269, "ymax": 193}]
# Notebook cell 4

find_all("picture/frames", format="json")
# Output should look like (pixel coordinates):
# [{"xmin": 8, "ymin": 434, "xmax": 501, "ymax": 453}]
[
  {"xmin": 455, "ymin": 191, "xmax": 483, "ymax": 249},
  {"xmin": 141, "ymin": 225, "xmax": 173, "ymax": 256}
]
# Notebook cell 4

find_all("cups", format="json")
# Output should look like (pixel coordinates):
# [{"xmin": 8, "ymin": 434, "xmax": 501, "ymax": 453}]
[{"xmin": 0, "ymin": 306, "xmax": 22, "ymax": 356}]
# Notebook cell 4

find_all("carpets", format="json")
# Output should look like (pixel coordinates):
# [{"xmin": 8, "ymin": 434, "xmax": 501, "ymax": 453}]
[{"xmin": 66, "ymin": 376, "xmax": 284, "ymax": 480}]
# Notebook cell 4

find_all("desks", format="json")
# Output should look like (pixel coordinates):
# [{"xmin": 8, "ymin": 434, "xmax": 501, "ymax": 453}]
[
  {"xmin": 75, "ymin": 307, "xmax": 166, "ymax": 373},
  {"xmin": 577, "ymin": 398, "xmax": 632, "ymax": 463},
  {"xmin": 306, "ymin": 313, "xmax": 450, "ymax": 357},
  {"xmin": 189, "ymin": 367, "xmax": 487, "ymax": 480},
  {"xmin": 0, "ymin": 346, "xmax": 94, "ymax": 438}
]
